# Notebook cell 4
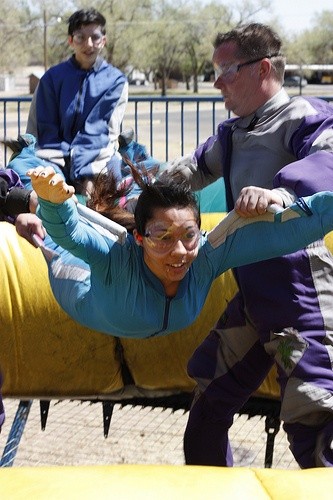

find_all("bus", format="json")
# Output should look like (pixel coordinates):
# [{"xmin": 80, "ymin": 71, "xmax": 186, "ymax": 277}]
[{"xmin": 282, "ymin": 64, "xmax": 333, "ymax": 102}]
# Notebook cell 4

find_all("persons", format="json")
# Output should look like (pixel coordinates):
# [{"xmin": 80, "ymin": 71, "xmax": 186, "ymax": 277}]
[
  {"xmin": 6, "ymin": 8, "xmax": 126, "ymax": 198},
  {"xmin": 26, "ymin": 157, "xmax": 333, "ymax": 339},
  {"xmin": 0, "ymin": 167, "xmax": 47, "ymax": 247},
  {"xmin": 156, "ymin": 21, "xmax": 333, "ymax": 469}
]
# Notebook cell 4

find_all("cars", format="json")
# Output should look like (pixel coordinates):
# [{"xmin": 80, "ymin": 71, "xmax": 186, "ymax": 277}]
[{"xmin": 126, "ymin": 72, "xmax": 149, "ymax": 86}]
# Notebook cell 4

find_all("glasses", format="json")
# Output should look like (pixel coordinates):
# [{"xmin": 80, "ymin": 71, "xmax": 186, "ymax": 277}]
[
  {"xmin": 69, "ymin": 31, "xmax": 103, "ymax": 46},
  {"xmin": 212, "ymin": 49, "xmax": 280, "ymax": 84},
  {"xmin": 138, "ymin": 216, "xmax": 201, "ymax": 253}
]
